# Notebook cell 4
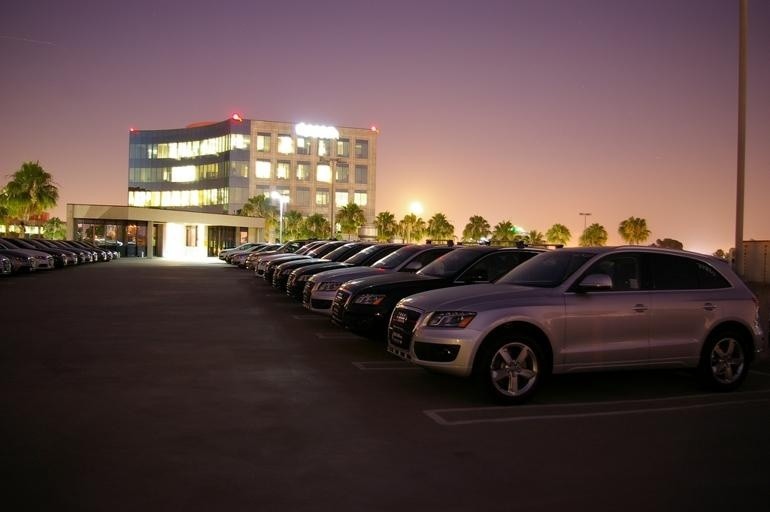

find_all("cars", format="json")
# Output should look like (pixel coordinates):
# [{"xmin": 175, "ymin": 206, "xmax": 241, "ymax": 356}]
[
  {"xmin": 0, "ymin": 237, "xmax": 121, "ymax": 274},
  {"xmin": 387, "ymin": 244, "xmax": 764, "ymax": 407},
  {"xmin": 327, "ymin": 239, "xmax": 569, "ymax": 337},
  {"xmin": 285, "ymin": 242, "xmax": 384, "ymax": 298},
  {"xmin": 218, "ymin": 241, "xmax": 342, "ymax": 291},
  {"xmin": 301, "ymin": 243, "xmax": 463, "ymax": 316}
]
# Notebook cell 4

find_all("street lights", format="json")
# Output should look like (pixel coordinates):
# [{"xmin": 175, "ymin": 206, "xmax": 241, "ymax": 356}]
[
  {"xmin": 271, "ymin": 191, "xmax": 289, "ymax": 243},
  {"xmin": 320, "ymin": 156, "xmax": 347, "ymax": 239},
  {"xmin": 579, "ymin": 212, "xmax": 590, "ymax": 225}
]
